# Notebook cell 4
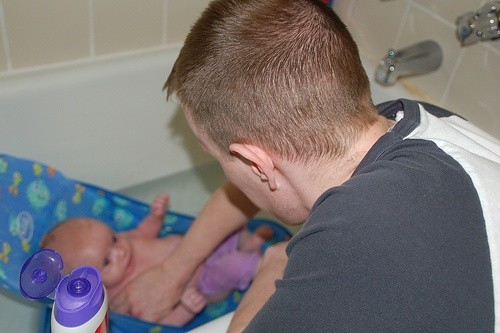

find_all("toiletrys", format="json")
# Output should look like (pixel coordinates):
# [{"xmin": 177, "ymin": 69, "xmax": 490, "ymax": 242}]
[{"xmin": 18, "ymin": 247, "xmax": 109, "ymax": 333}]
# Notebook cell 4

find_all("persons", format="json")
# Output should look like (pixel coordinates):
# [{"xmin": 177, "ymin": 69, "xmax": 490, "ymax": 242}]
[
  {"xmin": 110, "ymin": 0, "xmax": 500, "ymax": 332},
  {"xmin": 40, "ymin": 193, "xmax": 274, "ymax": 327}
]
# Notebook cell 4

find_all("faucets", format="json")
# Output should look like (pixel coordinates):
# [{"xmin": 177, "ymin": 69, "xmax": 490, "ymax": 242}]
[{"xmin": 373, "ymin": 37, "xmax": 444, "ymax": 87}]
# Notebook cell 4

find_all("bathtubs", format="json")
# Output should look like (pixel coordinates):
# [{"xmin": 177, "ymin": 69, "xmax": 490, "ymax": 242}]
[{"xmin": 1, "ymin": 42, "xmax": 500, "ymax": 333}]
